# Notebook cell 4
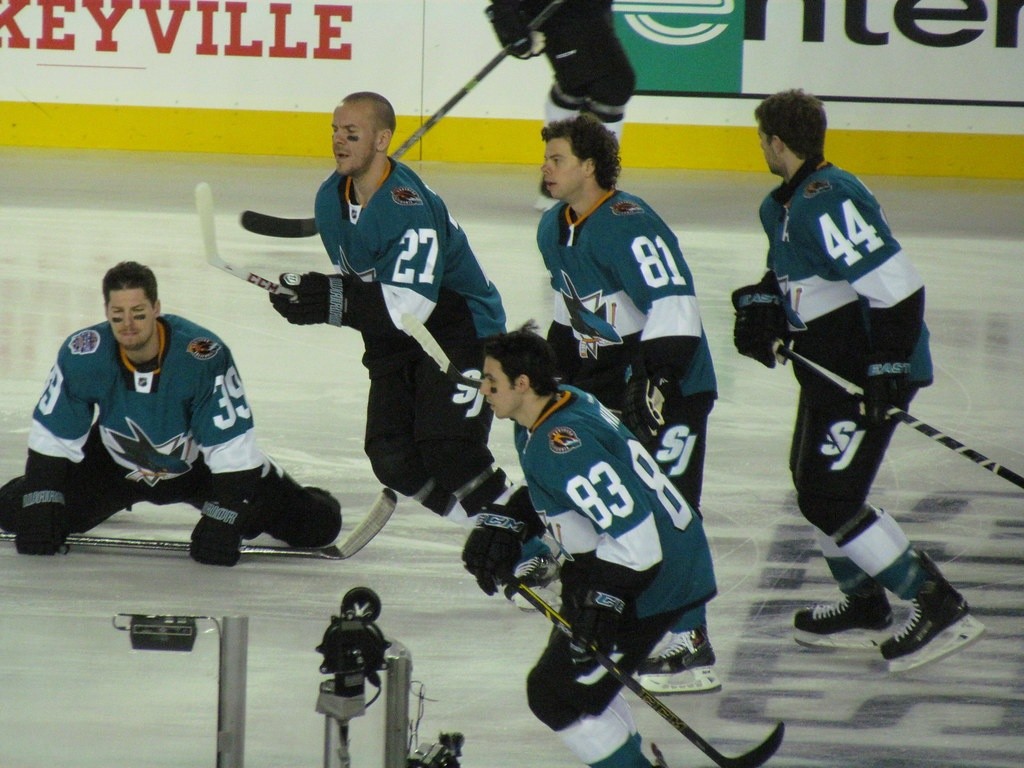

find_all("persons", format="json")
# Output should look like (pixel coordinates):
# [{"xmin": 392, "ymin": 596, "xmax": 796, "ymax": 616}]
[
  {"xmin": 537, "ymin": 116, "xmax": 718, "ymax": 695},
  {"xmin": 0, "ymin": 261, "xmax": 342, "ymax": 566},
  {"xmin": 463, "ymin": 331, "xmax": 719, "ymax": 768},
  {"xmin": 269, "ymin": 92, "xmax": 561, "ymax": 610},
  {"xmin": 485, "ymin": 1, "xmax": 637, "ymax": 211},
  {"xmin": 735, "ymin": 92, "xmax": 987, "ymax": 672}
]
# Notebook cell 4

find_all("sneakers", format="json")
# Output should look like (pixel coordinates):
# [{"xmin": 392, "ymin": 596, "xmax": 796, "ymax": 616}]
[
  {"xmin": 534, "ymin": 176, "xmax": 560, "ymax": 212},
  {"xmin": 792, "ymin": 582, "xmax": 895, "ymax": 650},
  {"xmin": 880, "ymin": 548, "xmax": 985, "ymax": 673},
  {"xmin": 512, "ymin": 545, "xmax": 562, "ymax": 611},
  {"xmin": 638, "ymin": 626, "xmax": 723, "ymax": 695}
]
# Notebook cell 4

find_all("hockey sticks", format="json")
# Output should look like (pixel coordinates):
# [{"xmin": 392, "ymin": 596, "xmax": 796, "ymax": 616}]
[
  {"xmin": 193, "ymin": 180, "xmax": 297, "ymax": 301},
  {"xmin": 399, "ymin": 312, "xmax": 481, "ymax": 388},
  {"xmin": 506, "ymin": 575, "xmax": 786, "ymax": 768},
  {"xmin": 774, "ymin": 342, "xmax": 1024, "ymax": 490},
  {"xmin": 240, "ymin": 43, "xmax": 513, "ymax": 239},
  {"xmin": 0, "ymin": 488, "xmax": 399, "ymax": 561}
]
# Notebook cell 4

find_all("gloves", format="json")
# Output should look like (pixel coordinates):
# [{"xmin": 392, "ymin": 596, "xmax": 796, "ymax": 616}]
[
  {"xmin": 14, "ymin": 506, "xmax": 71, "ymax": 556},
  {"xmin": 484, "ymin": 0, "xmax": 547, "ymax": 59},
  {"xmin": 850, "ymin": 357, "xmax": 910, "ymax": 430},
  {"xmin": 731, "ymin": 284, "xmax": 791, "ymax": 369},
  {"xmin": 459, "ymin": 505, "xmax": 527, "ymax": 598},
  {"xmin": 190, "ymin": 488, "xmax": 241, "ymax": 567},
  {"xmin": 270, "ymin": 271, "xmax": 358, "ymax": 328},
  {"xmin": 620, "ymin": 366, "xmax": 672, "ymax": 446},
  {"xmin": 569, "ymin": 589, "xmax": 625, "ymax": 664}
]
